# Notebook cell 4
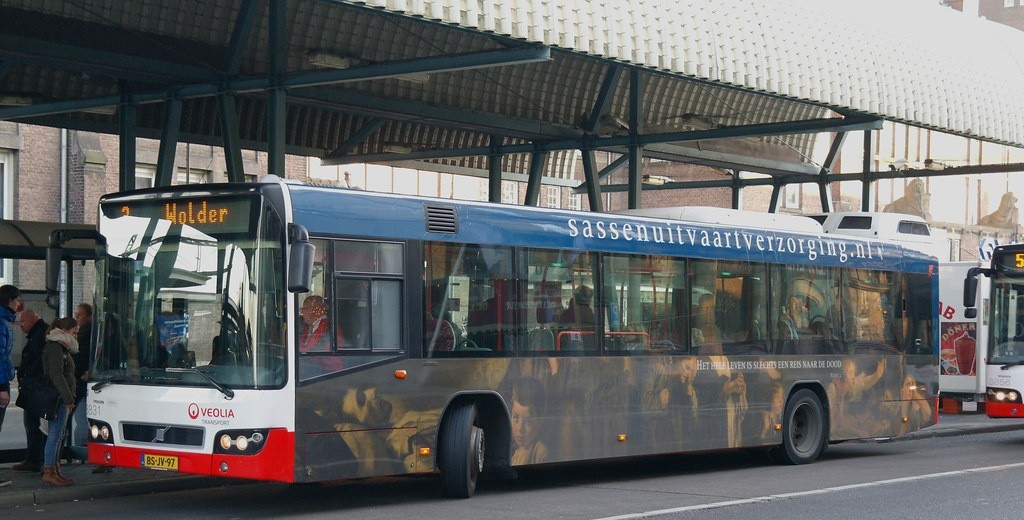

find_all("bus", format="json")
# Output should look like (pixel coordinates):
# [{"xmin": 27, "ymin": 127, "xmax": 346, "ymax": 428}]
[
  {"xmin": 44, "ymin": 174, "xmax": 943, "ymax": 499},
  {"xmin": 963, "ymin": 241, "xmax": 1024, "ymax": 419}
]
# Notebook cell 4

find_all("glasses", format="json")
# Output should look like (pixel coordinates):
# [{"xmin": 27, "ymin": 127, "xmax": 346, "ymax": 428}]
[{"xmin": 301, "ymin": 307, "xmax": 313, "ymax": 314}]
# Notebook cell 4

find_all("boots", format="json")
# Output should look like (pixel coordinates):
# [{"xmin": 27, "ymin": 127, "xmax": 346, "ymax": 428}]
[
  {"xmin": 55, "ymin": 463, "xmax": 73, "ymax": 483},
  {"xmin": 43, "ymin": 465, "xmax": 71, "ymax": 485}
]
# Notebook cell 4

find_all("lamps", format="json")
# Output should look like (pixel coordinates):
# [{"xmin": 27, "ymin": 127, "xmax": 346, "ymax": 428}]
[
  {"xmin": 923, "ymin": 158, "xmax": 960, "ymax": 172},
  {"xmin": 599, "ymin": 112, "xmax": 630, "ymax": 131},
  {"xmin": 384, "ymin": 141, "xmax": 444, "ymax": 155},
  {"xmin": 306, "ymin": 47, "xmax": 368, "ymax": 69},
  {"xmin": 80, "ymin": 105, "xmax": 117, "ymax": 114},
  {"xmin": 395, "ymin": 73, "xmax": 432, "ymax": 85},
  {"xmin": 680, "ymin": 113, "xmax": 721, "ymax": 132},
  {"xmin": 642, "ymin": 157, "xmax": 675, "ymax": 185},
  {"xmin": 1, "ymin": 95, "xmax": 50, "ymax": 106}
]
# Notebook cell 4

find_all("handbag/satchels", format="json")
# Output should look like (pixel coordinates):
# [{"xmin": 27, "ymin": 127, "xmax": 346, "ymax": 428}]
[{"xmin": 15, "ymin": 383, "xmax": 61, "ymax": 420}]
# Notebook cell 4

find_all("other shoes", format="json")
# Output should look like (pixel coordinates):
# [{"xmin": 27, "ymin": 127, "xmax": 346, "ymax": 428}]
[
  {"xmin": 29, "ymin": 463, "xmax": 40, "ymax": 471},
  {"xmin": 13, "ymin": 459, "xmax": 32, "ymax": 470},
  {"xmin": 92, "ymin": 464, "xmax": 112, "ymax": 472},
  {"xmin": 0, "ymin": 478, "xmax": 12, "ymax": 486}
]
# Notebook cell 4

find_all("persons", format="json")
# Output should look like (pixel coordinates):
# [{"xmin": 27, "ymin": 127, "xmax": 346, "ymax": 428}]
[
  {"xmin": 464, "ymin": 277, "xmax": 859, "ymax": 356},
  {"xmin": 0, "ymin": 284, "xmax": 117, "ymax": 487},
  {"xmin": 297, "ymin": 358, "xmax": 932, "ymax": 475},
  {"xmin": 299, "ymin": 296, "xmax": 347, "ymax": 373},
  {"xmin": 208, "ymin": 335, "xmax": 237, "ymax": 365}
]
